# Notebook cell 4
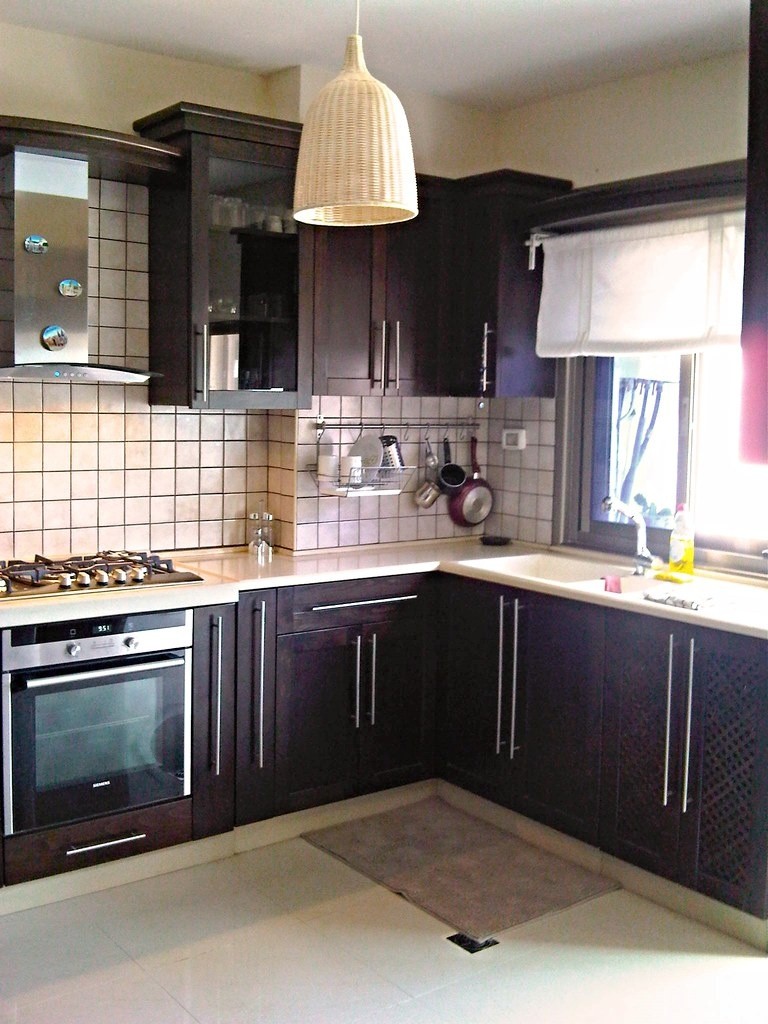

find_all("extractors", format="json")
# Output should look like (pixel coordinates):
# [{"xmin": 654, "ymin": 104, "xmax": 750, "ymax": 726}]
[{"xmin": 0, "ymin": 152, "xmax": 164, "ymax": 385}]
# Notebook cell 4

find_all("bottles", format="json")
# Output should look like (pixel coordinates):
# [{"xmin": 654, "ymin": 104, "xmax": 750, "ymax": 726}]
[{"xmin": 247, "ymin": 512, "xmax": 274, "ymax": 555}]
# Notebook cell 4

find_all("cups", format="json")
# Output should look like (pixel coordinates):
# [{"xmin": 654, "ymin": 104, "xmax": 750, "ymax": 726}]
[
  {"xmin": 206, "ymin": 193, "xmax": 298, "ymax": 319},
  {"xmin": 414, "ymin": 480, "xmax": 442, "ymax": 509},
  {"xmin": 340, "ymin": 456, "xmax": 362, "ymax": 484},
  {"xmin": 317, "ymin": 455, "xmax": 339, "ymax": 482}
]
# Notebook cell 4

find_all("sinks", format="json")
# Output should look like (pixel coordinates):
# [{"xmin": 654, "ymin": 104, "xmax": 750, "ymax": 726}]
[
  {"xmin": 565, "ymin": 576, "xmax": 673, "ymax": 595},
  {"xmin": 458, "ymin": 553, "xmax": 634, "ymax": 583}
]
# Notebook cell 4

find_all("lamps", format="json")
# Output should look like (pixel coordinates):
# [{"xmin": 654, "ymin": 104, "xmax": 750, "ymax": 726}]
[{"xmin": 291, "ymin": 0, "xmax": 419, "ymax": 227}]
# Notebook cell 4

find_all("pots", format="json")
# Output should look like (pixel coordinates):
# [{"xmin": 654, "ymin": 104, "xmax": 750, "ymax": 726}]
[
  {"xmin": 447, "ymin": 437, "xmax": 494, "ymax": 527},
  {"xmin": 437, "ymin": 438, "xmax": 467, "ymax": 497}
]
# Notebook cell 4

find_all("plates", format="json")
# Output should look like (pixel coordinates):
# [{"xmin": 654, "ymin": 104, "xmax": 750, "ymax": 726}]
[
  {"xmin": 479, "ymin": 537, "xmax": 510, "ymax": 545},
  {"xmin": 347, "ymin": 435, "xmax": 383, "ymax": 489}
]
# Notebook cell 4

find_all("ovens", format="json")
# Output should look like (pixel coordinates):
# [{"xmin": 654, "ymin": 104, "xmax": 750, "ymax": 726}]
[{"xmin": 0, "ymin": 603, "xmax": 239, "ymax": 887}]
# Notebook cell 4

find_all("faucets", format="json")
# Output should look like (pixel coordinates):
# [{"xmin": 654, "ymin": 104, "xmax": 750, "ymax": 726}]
[{"xmin": 602, "ymin": 496, "xmax": 663, "ymax": 569}]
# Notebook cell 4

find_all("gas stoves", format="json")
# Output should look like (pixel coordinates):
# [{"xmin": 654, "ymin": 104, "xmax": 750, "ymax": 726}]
[{"xmin": 0, "ymin": 550, "xmax": 238, "ymax": 629}]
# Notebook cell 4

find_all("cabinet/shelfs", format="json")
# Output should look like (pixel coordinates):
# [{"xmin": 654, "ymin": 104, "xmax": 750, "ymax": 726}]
[
  {"xmin": 434, "ymin": 572, "xmax": 768, "ymax": 922},
  {"xmin": 150, "ymin": 132, "xmax": 575, "ymax": 409},
  {"xmin": 193, "ymin": 570, "xmax": 445, "ymax": 838}
]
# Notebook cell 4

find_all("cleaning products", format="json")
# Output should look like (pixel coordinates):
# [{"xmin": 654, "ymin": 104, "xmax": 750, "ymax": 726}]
[{"xmin": 669, "ymin": 503, "xmax": 695, "ymax": 577}]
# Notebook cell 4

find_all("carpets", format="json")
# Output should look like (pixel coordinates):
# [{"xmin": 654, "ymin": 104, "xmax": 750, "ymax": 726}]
[{"xmin": 299, "ymin": 795, "xmax": 622, "ymax": 942}]
